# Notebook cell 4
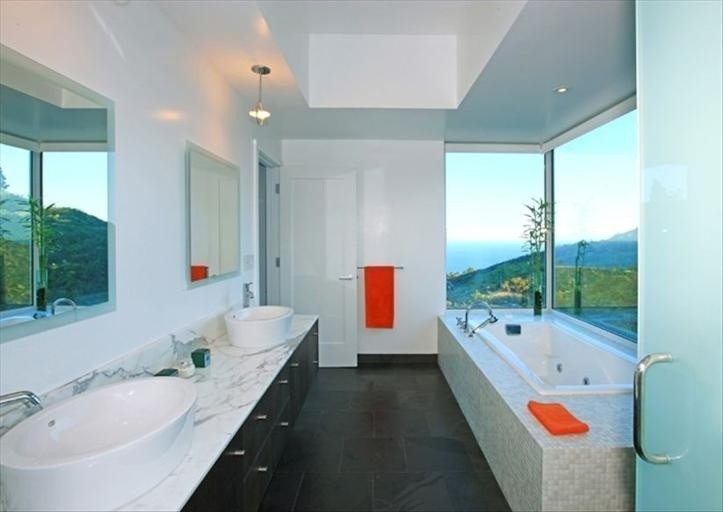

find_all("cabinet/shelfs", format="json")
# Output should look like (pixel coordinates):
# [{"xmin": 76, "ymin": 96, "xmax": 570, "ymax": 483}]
[{"xmin": 176, "ymin": 317, "xmax": 317, "ymax": 511}]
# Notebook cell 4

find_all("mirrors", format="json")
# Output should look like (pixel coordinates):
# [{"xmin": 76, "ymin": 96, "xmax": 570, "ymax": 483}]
[
  {"xmin": 0, "ymin": 43, "xmax": 116, "ymax": 341},
  {"xmin": 183, "ymin": 140, "xmax": 241, "ymax": 291}
]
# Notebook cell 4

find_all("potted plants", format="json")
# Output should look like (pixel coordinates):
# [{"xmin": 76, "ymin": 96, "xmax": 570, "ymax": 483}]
[
  {"xmin": 13, "ymin": 195, "xmax": 71, "ymax": 312},
  {"xmin": 519, "ymin": 197, "xmax": 558, "ymax": 316},
  {"xmin": 574, "ymin": 239, "xmax": 589, "ymax": 313}
]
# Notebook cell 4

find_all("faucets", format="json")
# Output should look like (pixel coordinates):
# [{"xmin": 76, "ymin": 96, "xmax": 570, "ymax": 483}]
[
  {"xmin": 0, "ymin": 390, "xmax": 41, "ymax": 408},
  {"xmin": 243, "ymin": 282, "xmax": 254, "ymax": 307},
  {"xmin": 464, "ymin": 300, "xmax": 494, "ymax": 331}
]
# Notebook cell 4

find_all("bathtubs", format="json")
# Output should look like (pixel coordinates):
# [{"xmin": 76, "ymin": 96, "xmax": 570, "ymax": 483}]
[{"xmin": 468, "ymin": 316, "xmax": 637, "ymax": 395}]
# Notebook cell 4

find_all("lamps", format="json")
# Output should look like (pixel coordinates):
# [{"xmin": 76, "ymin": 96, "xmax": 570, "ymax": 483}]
[{"xmin": 248, "ymin": 64, "xmax": 271, "ymax": 127}]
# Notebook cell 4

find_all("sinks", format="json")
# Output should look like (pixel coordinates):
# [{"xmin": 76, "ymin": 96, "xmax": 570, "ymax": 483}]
[
  {"xmin": 0, "ymin": 375, "xmax": 197, "ymax": 512},
  {"xmin": 225, "ymin": 305, "xmax": 294, "ymax": 352}
]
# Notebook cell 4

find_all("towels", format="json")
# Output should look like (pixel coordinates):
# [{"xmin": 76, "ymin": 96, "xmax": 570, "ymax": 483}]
[
  {"xmin": 363, "ymin": 266, "xmax": 394, "ymax": 328},
  {"xmin": 527, "ymin": 401, "xmax": 589, "ymax": 435}
]
